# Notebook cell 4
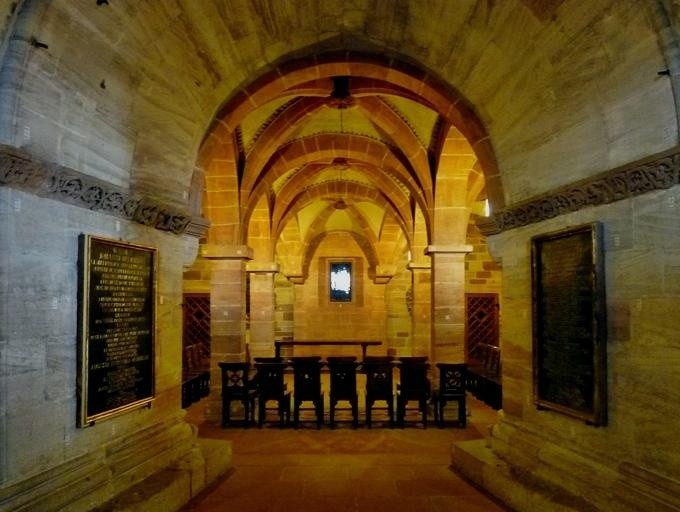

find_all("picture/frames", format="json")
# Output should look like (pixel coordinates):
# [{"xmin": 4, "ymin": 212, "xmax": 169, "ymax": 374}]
[
  {"xmin": 530, "ymin": 218, "xmax": 606, "ymax": 428},
  {"xmin": 75, "ymin": 233, "xmax": 158, "ymax": 429}
]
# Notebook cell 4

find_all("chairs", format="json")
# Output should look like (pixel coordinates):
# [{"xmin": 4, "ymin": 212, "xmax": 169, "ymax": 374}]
[
  {"xmin": 182, "ymin": 342, "xmax": 211, "ymax": 409},
  {"xmin": 468, "ymin": 341, "xmax": 503, "ymax": 411},
  {"xmin": 219, "ymin": 356, "xmax": 468, "ymax": 430}
]
